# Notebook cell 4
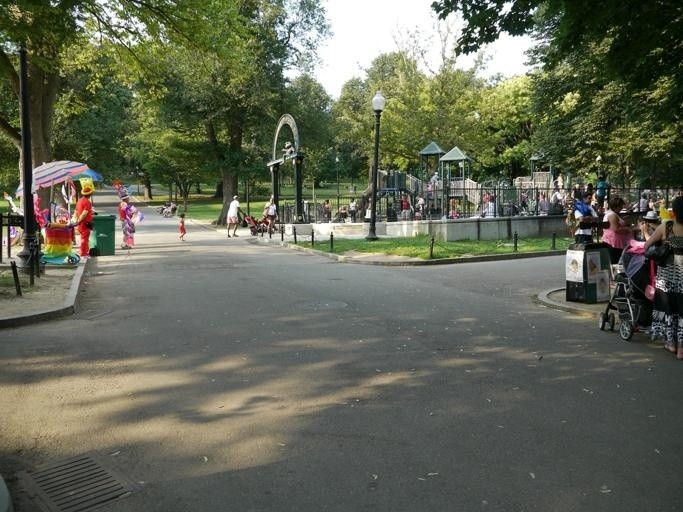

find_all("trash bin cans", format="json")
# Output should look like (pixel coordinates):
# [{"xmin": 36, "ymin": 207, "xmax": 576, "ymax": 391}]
[
  {"xmin": 88, "ymin": 214, "xmax": 116, "ymax": 256},
  {"xmin": 565, "ymin": 241, "xmax": 611, "ymax": 303}
]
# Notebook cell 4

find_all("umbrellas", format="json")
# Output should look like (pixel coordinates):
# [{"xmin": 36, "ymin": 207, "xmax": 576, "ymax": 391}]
[
  {"xmin": 14, "ymin": 159, "xmax": 88, "ymax": 197},
  {"xmin": 68, "ymin": 168, "xmax": 101, "ymax": 183}
]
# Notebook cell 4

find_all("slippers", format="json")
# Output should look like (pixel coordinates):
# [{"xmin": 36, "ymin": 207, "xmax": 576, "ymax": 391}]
[{"xmin": 664, "ymin": 344, "xmax": 677, "ymax": 353}]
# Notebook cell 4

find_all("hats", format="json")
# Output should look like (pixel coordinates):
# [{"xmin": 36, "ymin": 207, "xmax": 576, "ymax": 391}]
[
  {"xmin": 119, "ymin": 189, "xmax": 129, "ymax": 200},
  {"xmin": 233, "ymin": 196, "xmax": 240, "ymax": 199},
  {"xmin": 642, "ymin": 211, "xmax": 660, "ymax": 220},
  {"xmin": 80, "ymin": 186, "xmax": 93, "ymax": 195}
]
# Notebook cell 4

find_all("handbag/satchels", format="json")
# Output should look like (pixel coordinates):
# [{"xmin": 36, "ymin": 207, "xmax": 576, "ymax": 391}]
[
  {"xmin": 565, "ymin": 208, "xmax": 580, "ymax": 234},
  {"xmin": 653, "ymin": 221, "xmax": 670, "ymax": 268},
  {"xmin": 85, "ymin": 221, "xmax": 94, "ymax": 230},
  {"xmin": 644, "ymin": 284, "xmax": 656, "ymax": 301},
  {"xmin": 262, "ymin": 206, "xmax": 269, "ymax": 218}
]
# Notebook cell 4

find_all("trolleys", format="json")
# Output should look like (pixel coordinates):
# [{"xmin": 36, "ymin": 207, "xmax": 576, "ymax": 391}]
[{"xmin": 40, "ymin": 222, "xmax": 81, "ymax": 265}]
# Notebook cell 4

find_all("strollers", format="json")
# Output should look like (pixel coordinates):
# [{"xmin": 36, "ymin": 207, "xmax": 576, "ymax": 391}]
[
  {"xmin": 245, "ymin": 216, "xmax": 274, "ymax": 236},
  {"xmin": 597, "ymin": 239, "xmax": 654, "ymax": 341}
]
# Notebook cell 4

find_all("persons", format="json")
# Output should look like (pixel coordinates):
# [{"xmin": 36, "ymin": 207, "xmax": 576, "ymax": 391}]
[
  {"xmin": 387, "ymin": 191, "xmax": 429, "ymax": 220},
  {"xmin": 324, "ymin": 200, "xmax": 331, "ymax": 222},
  {"xmin": 111, "ymin": 180, "xmax": 137, "ymax": 249},
  {"xmin": 264, "ymin": 197, "xmax": 276, "ymax": 233},
  {"xmin": 159, "ymin": 202, "xmax": 169, "ymax": 214},
  {"xmin": 483, "ymin": 195, "xmax": 496, "ymax": 216},
  {"xmin": 550, "ymin": 189, "xmax": 564, "ymax": 205},
  {"xmin": 177, "ymin": 214, "xmax": 186, "ymax": 240},
  {"xmin": 339, "ymin": 206, "xmax": 347, "ymax": 223},
  {"xmin": 163, "ymin": 203, "xmax": 176, "ymax": 217},
  {"xmin": 479, "ymin": 191, "xmax": 490, "ymax": 210},
  {"xmin": 72, "ymin": 188, "xmax": 93, "ymax": 259},
  {"xmin": 349, "ymin": 198, "xmax": 356, "ymax": 209},
  {"xmin": 564, "ymin": 175, "xmax": 683, "ymax": 360},
  {"xmin": 427, "ymin": 181, "xmax": 433, "ymax": 199},
  {"xmin": 430, "ymin": 171, "xmax": 440, "ymax": 183},
  {"xmin": 226, "ymin": 195, "xmax": 244, "ymax": 237}
]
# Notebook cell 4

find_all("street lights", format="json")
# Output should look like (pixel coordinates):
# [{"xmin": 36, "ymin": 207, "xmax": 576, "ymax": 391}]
[
  {"xmin": 365, "ymin": 90, "xmax": 386, "ymax": 241},
  {"xmin": 336, "ymin": 156, "xmax": 339, "ymax": 222}
]
